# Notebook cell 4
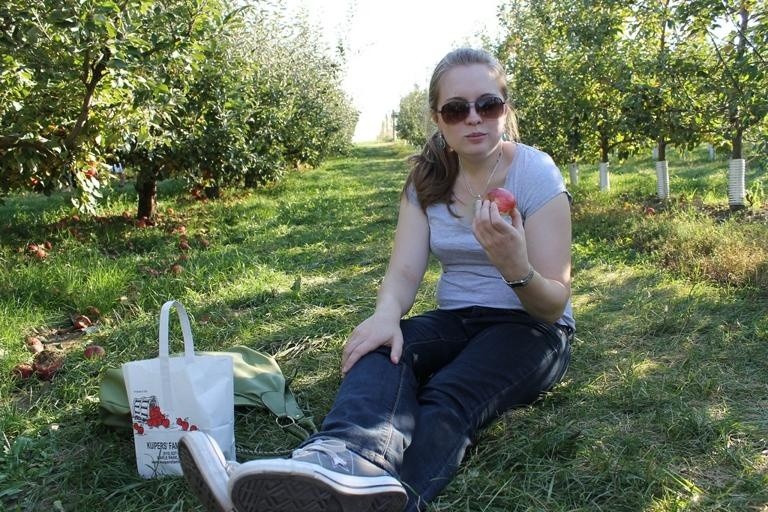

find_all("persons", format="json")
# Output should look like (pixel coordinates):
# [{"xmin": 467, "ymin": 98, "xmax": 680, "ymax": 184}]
[{"xmin": 177, "ymin": 50, "xmax": 572, "ymax": 512}]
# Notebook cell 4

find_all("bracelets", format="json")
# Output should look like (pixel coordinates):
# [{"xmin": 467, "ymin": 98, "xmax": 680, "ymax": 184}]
[{"xmin": 499, "ymin": 261, "xmax": 535, "ymax": 287}]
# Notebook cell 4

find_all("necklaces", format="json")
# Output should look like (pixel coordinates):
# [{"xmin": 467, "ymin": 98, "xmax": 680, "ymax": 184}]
[{"xmin": 454, "ymin": 139, "xmax": 503, "ymax": 201}]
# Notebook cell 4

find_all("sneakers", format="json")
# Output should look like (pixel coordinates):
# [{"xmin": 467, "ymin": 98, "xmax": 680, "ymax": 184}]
[{"xmin": 178, "ymin": 430, "xmax": 410, "ymax": 512}]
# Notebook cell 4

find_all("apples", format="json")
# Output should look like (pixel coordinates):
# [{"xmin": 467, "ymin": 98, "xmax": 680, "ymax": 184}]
[
  {"xmin": 170, "ymin": 263, "xmax": 182, "ymax": 273},
  {"xmin": 133, "ymin": 405, "xmax": 196, "ymax": 435},
  {"xmin": 201, "ymin": 238, "xmax": 210, "ymax": 246},
  {"xmin": 178, "ymin": 240, "xmax": 191, "ymax": 261},
  {"xmin": 73, "ymin": 305, "xmax": 100, "ymax": 331},
  {"xmin": 646, "ymin": 207, "xmax": 654, "ymax": 215},
  {"xmin": 26, "ymin": 241, "xmax": 52, "ymax": 261},
  {"xmin": 11, "ymin": 334, "xmax": 65, "ymax": 388},
  {"xmin": 28, "ymin": 176, "xmax": 39, "ymax": 187},
  {"xmin": 84, "ymin": 160, "xmax": 96, "ymax": 176},
  {"xmin": 122, "ymin": 206, "xmax": 187, "ymax": 234},
  {"xmin": 83, "ymin": 346, "xmax": 105, "ymax": 359},
  {"xmin": 488, "ymin": 188, "xmax": 517, "ymax": 216}
]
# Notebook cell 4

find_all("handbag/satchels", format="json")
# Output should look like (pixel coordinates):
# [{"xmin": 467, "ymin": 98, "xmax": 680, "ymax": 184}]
[
  {"xmin": 98, "ymin": 343, "xmax": 319, "ymax": 459},
  {"xmin": 121, "ymin": 301, "xmax": 236, "ymax": 480}
]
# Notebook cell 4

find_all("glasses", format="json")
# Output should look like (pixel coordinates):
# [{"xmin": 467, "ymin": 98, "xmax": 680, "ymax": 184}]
[{"xmin": 430, "ymin": 95, "xmax": 508, "ymax": 125}]
